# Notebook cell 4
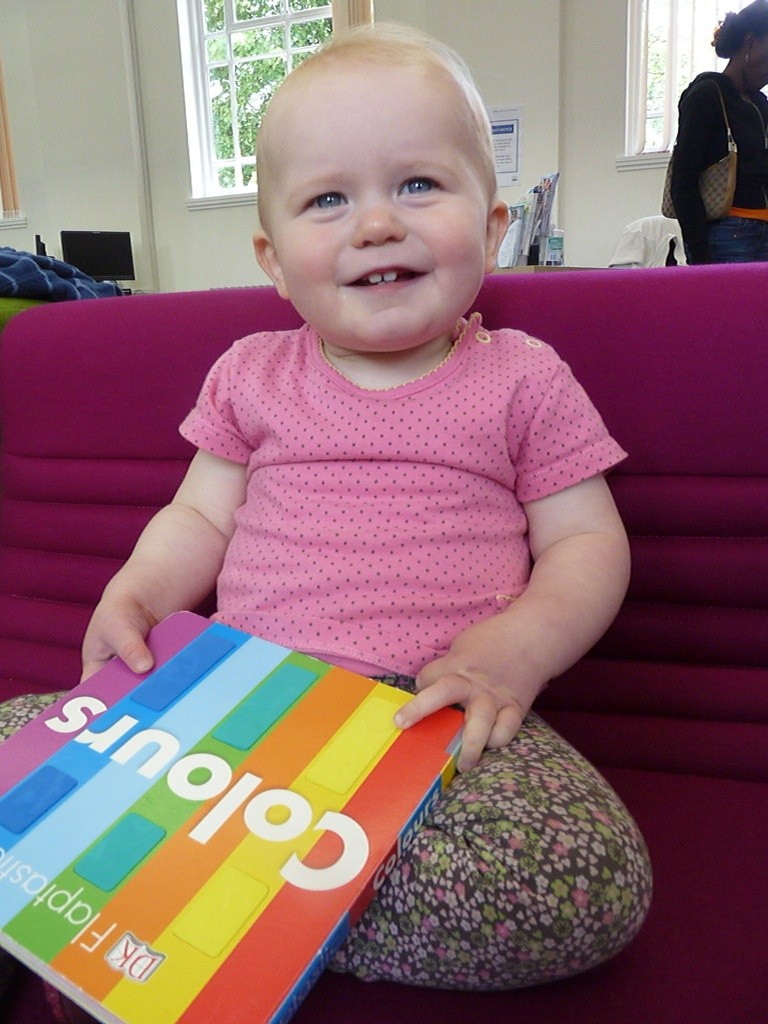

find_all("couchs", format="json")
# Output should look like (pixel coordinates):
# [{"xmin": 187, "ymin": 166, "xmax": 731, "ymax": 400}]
[{"xmin": 0, "ymin": 263, "xmax": 767, "ymax": 1024}]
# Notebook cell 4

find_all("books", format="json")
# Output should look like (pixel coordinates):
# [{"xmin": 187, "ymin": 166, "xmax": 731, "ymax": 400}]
[
  {"xmin": 500, "ymin": 174, "xmax": 560, "ymax": 267},
  {"xmin": 0, "ymin": 608, "xmax": 465, "ymax": 1024}
]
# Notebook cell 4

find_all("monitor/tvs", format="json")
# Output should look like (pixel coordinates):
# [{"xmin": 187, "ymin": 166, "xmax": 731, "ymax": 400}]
[{"xmin": 60, "ymin": 231, "xmax": 136, "ymax": 281}]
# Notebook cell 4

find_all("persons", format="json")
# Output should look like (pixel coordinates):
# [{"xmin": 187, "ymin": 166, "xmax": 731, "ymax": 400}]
[
  {"xmin": 670, "ymin": 1, "xmax": 768, "ymax": 264},
  {"xmin": 0, "ymin": 28, "xmax": 651, "ymax": 991}
]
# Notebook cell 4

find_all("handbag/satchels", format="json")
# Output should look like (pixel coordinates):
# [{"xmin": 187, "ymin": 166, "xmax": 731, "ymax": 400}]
[{"xmin": 663, "ymin": 77, "xmax": 738, "ymax": 222}]
